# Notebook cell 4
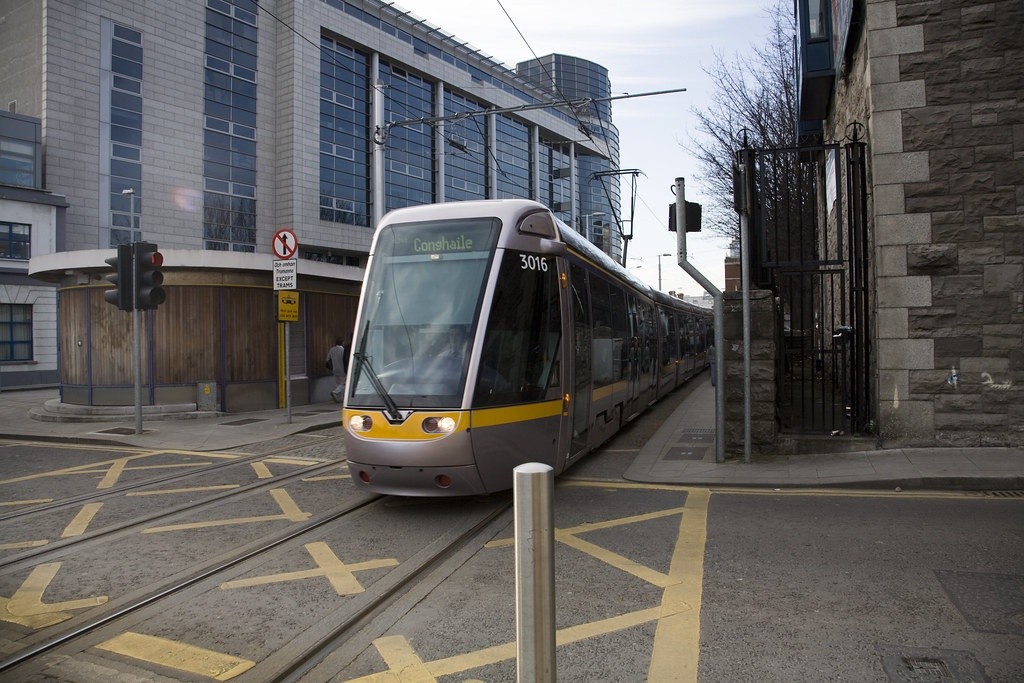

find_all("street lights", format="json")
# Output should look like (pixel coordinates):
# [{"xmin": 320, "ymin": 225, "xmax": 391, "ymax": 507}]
[{"xmin": 122, "ymin": 188, "xmax": 142, "ymax": 434}]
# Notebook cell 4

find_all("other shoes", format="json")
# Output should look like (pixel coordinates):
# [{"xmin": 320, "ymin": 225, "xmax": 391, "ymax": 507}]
[
  {"xmin": 330, "ymin": 391, "xmax": 339, "ymax": 404},
  {"xmin": 325, "ymin": 358, "xmax": 333, "ymax": 371}
]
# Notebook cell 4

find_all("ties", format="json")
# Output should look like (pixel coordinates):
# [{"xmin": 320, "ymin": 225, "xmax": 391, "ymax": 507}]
[{"xmin": 454, "ymin": 351, "xmax": 459, "ymax": 358}]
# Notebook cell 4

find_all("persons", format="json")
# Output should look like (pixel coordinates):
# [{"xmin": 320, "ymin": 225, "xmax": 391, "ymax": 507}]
[
  {"xmin": 630, "ymin": 316, "xmax": 714, "ymax": 381},
  {"xmin": 437, "ymin": 324, "xmax": 468, "ymax": 362},
  {"xmin": 707, "ymin": 323, "xmax": 714, "ymax": 349},
  {"xmin": 326, "ymin": 332, "xmax": 354, "ymax": 403},
  {"xmin": 708, "ymin": 337, "xmax": 716, "ymax": 386}
]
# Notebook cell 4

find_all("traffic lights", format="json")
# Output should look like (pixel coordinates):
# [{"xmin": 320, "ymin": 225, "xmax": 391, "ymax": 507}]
[
  {"xmin": 105, "ymin": 244, "xmax": 134, "ymax": 312},
  {"xmin": 133, "ymin": 241, "xmax": 166, "ymax": 311}
]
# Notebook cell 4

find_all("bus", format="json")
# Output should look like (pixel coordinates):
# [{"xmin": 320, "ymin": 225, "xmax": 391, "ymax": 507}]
[{"xmin": 339, "ymin": 167, "xmax": 715, "ymax": 498}]
